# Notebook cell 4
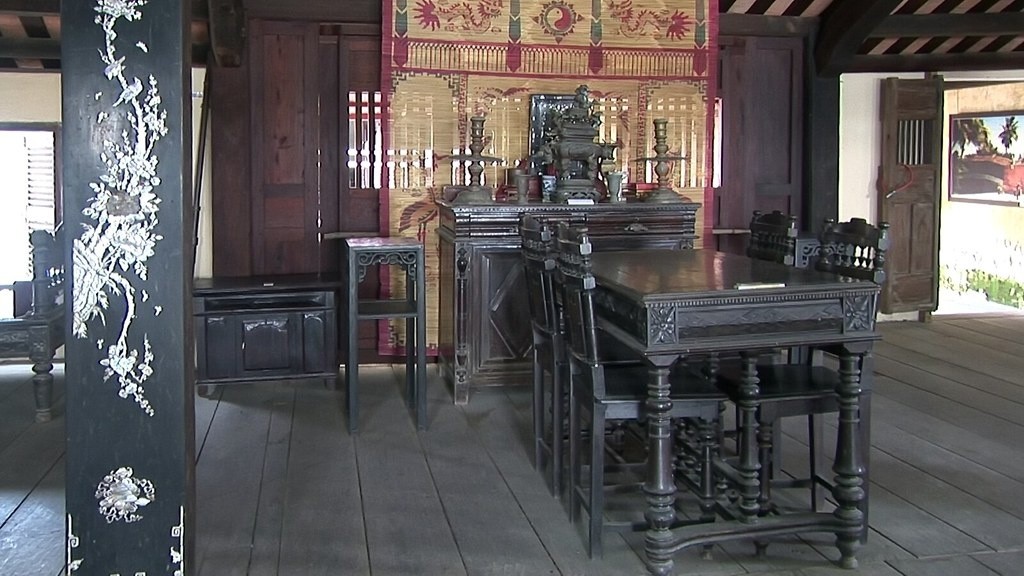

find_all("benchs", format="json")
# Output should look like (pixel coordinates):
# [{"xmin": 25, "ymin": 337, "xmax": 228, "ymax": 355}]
[{"xmin": 0, "ymin": 221, "xmax": 64, "ymax": 424}]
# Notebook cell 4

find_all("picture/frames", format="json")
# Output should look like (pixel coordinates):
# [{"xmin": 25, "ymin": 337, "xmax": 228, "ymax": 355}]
[{"xmin": 948, "ymin": 110, "xmax": 1024, "ymax": 208}]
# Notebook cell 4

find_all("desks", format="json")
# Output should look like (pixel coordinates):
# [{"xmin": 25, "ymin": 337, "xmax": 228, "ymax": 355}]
[{"xmin": 548, "ymin": 250, "xmax": 882, "ymax": 576}]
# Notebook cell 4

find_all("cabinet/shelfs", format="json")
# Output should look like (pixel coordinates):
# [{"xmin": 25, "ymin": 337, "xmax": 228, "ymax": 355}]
[
  {"xmin": 191, "ymin": 274, "xmax": 343, "ymax": 396},
  {"xmin": 436, "ymin": 199, "xmax": 703, "ymax": 410}
]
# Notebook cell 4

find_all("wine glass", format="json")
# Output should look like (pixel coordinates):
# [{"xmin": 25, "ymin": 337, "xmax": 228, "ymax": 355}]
[
  {"xmin": 606, "ymin": 173, "xmax": 622, "ymax": 203},
  {"xmin": 515, "ymin": 174, "xmax": 529, "ymax": 204}
]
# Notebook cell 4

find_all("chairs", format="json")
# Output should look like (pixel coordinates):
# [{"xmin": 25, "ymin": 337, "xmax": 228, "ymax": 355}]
[{"xmin": 517, "ymin": 211, "xmax": 890, "ymax": 559}]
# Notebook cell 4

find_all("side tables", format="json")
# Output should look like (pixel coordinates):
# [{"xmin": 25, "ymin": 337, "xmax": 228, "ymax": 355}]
[{"xmin": 343, "ymin": 236, "xmax": 429, "ymax": 436}]
